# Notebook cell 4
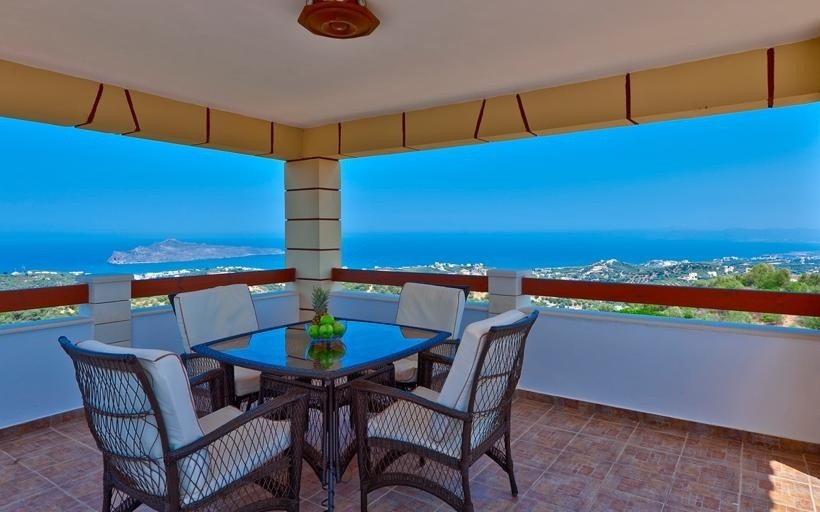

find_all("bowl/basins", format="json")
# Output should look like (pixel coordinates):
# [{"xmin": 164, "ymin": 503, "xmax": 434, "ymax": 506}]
[
  {"xmin": 303, "ymin": 320, "xmax": 346, "ymax": 341},
  {"xmin": 304, "ymin": 339, "xmax": 350, "ymax": 363}
]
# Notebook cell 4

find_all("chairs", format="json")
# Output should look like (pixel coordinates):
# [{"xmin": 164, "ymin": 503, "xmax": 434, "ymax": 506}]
[
  {"xmin": 373, "ymin": 280, "xmax": 469, "ymax": 406},
  {"xmin": 56, "ymin": 332, "xmax": 310, "ymax": 511},
  {"xmin": 349, "ymin": 304, "xmax": 541, "ymax": 511},
  {"xmin": 167, "ymin": 282, "xmax": 263, "ymax": 414}
]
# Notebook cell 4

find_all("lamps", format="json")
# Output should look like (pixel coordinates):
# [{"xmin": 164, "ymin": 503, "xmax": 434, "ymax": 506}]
[{"xmin": 295, "ymin": 1, "xmax": 382, "ymax": 40}]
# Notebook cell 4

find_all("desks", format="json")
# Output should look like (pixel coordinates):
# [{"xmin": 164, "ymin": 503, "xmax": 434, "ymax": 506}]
[{"xmin": 190, "ymin": 314, "xmax": 453, "ymax": 511}]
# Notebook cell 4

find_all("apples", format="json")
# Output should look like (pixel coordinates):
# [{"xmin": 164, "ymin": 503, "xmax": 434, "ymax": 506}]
[
  {"xmin": 308, "ymin": 340, "xmax": 346, "ymax": 369},
  {"xmin": 308, "ymin": 314, "xmax": 345, "ymax": 338}
]
